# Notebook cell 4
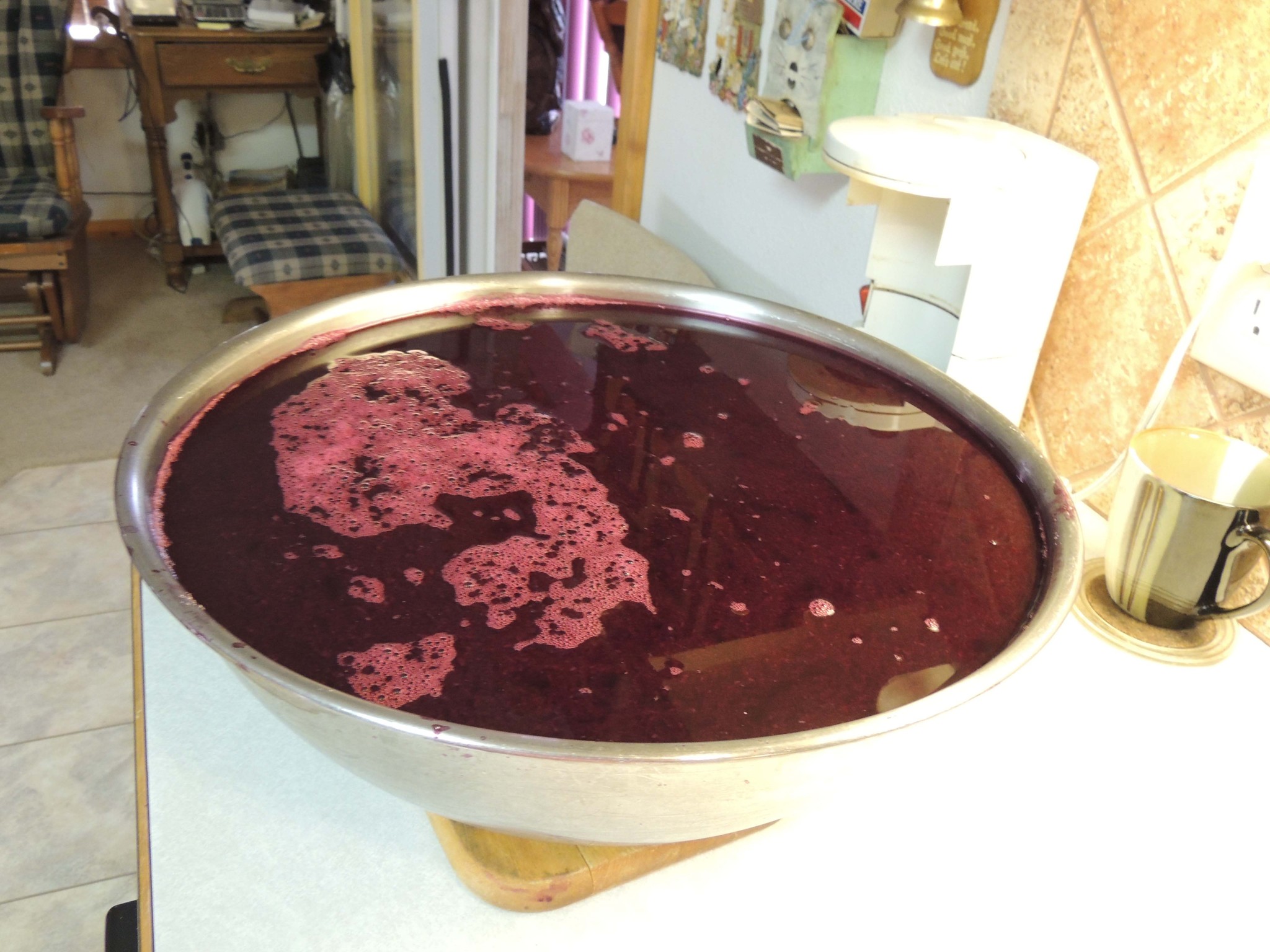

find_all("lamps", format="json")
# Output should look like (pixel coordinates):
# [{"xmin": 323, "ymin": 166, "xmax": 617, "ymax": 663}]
[{"xmin": 895, "ymin": 0, "xmax": 962, "ymax": 27}]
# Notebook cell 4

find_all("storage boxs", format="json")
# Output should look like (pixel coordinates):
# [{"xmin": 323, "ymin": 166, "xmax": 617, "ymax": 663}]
[{"xmin": 560, "ymin": 100, "xmax": 615, "ymax": 161}]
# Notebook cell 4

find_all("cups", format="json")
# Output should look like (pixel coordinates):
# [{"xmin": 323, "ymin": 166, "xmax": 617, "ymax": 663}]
[{"xmin": 1102, "ymin": 428, "xmax": 1270, "ymax": 629}]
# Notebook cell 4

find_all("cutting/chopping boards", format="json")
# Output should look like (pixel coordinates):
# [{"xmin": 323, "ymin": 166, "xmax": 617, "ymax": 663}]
[{"xmin": 424, "ymin": 809, "xmax": 781, "ymax": 917}]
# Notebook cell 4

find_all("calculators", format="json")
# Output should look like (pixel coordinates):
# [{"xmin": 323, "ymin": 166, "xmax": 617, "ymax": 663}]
[{"xmin": 193, "ymin": 0, "xmax": 246, "ymax": 21}]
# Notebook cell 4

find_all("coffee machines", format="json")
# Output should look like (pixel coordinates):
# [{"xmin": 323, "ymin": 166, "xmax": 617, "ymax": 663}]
[{"xmin": 823, "ymin": 116, "xmax": 1104, "ymax": 426}]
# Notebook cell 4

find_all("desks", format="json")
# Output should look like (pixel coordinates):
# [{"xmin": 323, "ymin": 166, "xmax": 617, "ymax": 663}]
[
  {"xmin": 121, "ymin": 29, "xmax": 338, "ymax": 293},
  {"xmin": 130, "ymin": 491, "xmax": 1270, "ymax": 952},
  {"xmin": 524, "ymin": 135, "xmax": 615, "ymax": 271}
]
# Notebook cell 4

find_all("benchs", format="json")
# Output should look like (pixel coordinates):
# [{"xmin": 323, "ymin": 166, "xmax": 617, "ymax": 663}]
[{"xmin": 206, "ymin": 193, "xmax": 407, "ymax": 323}]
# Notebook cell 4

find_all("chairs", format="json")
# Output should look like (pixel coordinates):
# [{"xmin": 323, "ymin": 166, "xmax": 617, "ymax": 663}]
[{"xmin": 0, "ymin": 106, "xmax": 92, "ymax": 377}]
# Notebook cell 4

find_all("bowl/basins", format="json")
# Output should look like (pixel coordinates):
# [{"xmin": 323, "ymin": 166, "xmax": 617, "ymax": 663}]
[{"xmin": 113, "ymin": 267, "xmax": 1086, "ymax": 850}]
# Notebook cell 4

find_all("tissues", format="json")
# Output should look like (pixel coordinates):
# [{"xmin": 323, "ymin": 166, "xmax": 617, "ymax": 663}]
[{"xmin": 548, "ymin": 97, "xmax": 615, "ymax": 162}]
